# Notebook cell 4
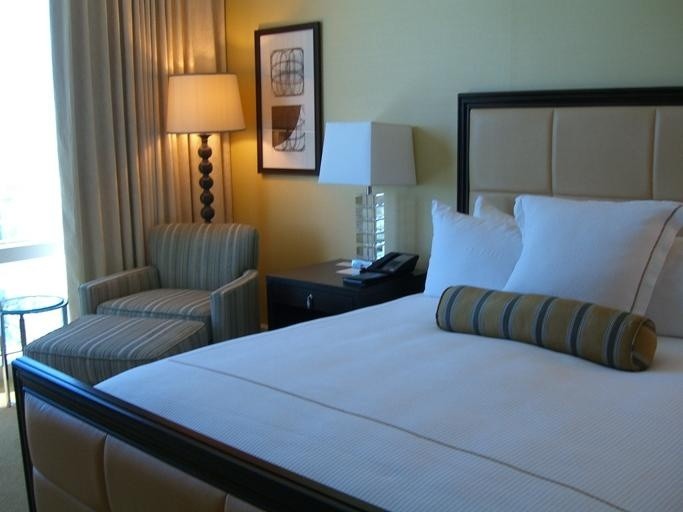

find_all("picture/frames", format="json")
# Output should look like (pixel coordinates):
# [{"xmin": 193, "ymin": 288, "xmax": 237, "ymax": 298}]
[{"xmin": 254, "ymin": 21, "xmax": 321, "ymax": 175}]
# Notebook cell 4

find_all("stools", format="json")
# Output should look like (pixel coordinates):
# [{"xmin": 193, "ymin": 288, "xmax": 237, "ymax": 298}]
[{"xmin": 23, "ymin": 315, "xmax": 209, "ymax": 384}]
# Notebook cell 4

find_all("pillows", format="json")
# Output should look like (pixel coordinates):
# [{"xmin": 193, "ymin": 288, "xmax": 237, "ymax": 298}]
[
  {"xmin": 436, "ymin": 285, "xmax": 658, "ymax": 372},
  {"xmin": 504, "ymin": 192, "xmax": 682, "ymax": 316},
  {"xmin": 644, "ymin": 235, "xmax": 682, "ymax": 337},
  {"xmin": 474, "ymin": 195, "xmax": 515, "ymax": 230},
  {"xmin": 424, "ymin": 200, "xmax": 522, "ymax": 308}
]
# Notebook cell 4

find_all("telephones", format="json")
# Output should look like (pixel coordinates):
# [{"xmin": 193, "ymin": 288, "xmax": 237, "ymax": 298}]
[{"xmin": 360, "ymin": 251, "xmax": 419, "ymax": 276}]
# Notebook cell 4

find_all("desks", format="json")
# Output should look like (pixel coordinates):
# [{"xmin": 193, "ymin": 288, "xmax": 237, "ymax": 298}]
[{"xmin": 0, "ymin": 294, "xmax": 67, "ymax": 406}]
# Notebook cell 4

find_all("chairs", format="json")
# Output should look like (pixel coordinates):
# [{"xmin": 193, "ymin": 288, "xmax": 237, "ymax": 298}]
[{"xmin": 79, "ymin": 222, "xmax": 259, "ymax": 340}]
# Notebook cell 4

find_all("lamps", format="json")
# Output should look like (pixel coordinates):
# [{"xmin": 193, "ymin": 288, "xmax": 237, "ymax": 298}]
[
  {"xmin": 316, "ymin": 120, "xmax": 418, "ymax": 265},
  {"xmin": 166, "ymin": 73, "xmax": 247, "ymax": 223}
]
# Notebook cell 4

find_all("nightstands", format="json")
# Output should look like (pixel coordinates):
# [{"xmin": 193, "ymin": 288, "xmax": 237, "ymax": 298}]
[{"xmin": 265, "ymin": 258, "xmax": 426, "ymax": 331}]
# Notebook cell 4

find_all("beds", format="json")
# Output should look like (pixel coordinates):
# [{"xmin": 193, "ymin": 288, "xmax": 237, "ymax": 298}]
[{"xmin": 14, "ymin": 288, "xmax": 682, "ymax": 512}]
